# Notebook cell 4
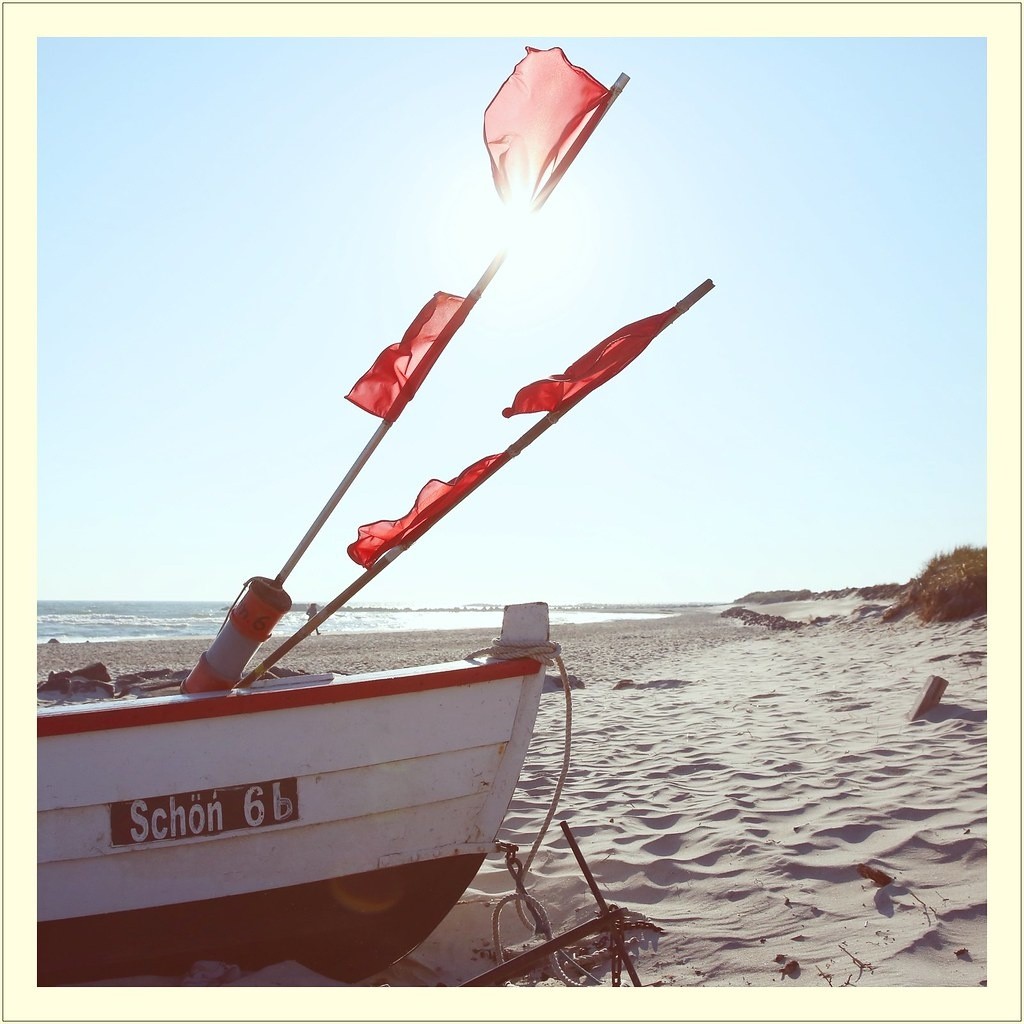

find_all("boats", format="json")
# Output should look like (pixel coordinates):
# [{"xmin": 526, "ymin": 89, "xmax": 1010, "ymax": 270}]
[{"xmin": 37, "ymin": 601, "xmax": 551, "ymax": 973}]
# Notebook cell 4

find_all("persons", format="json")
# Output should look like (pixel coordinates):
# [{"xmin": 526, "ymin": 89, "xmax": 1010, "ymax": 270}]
[
  {"xmin": 346, "ymin": 453, "xmax": 513, "ymax": 572},
  {"xmin": 306, "ymin": 603, "xmax": 321, "ymax": 636}
]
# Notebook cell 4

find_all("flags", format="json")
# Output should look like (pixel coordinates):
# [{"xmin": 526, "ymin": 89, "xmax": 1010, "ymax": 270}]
[
  {"xmin": 346, "ymin": 291, "xmax": 475, "ymax": 425},
  {"xmin": 484, "ymin": 47, "xmax": 610, "ymax": 208},
  {"xmin": 502, "ymin": 306, "xmax": 677, "ymax": 418}
]
